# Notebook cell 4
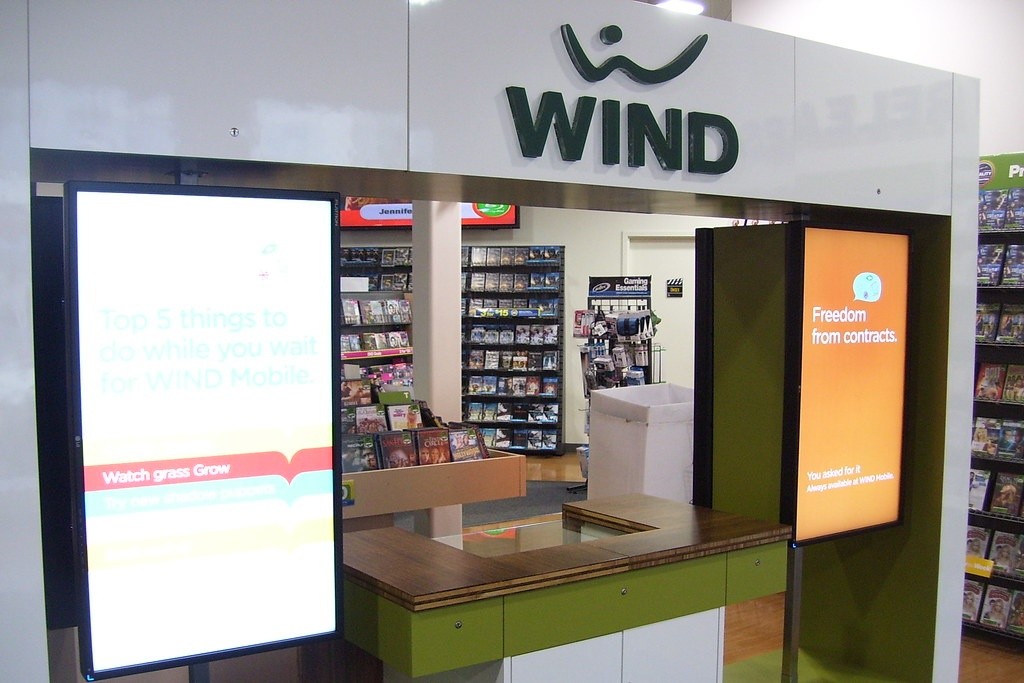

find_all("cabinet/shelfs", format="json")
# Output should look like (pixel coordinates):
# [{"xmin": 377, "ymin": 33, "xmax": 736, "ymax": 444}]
[
  {"xmin": 342, "ymin": 244, "xmax": 565, "ymax": 457},
  {"xmin": 963, "ymin": 155, "xmax": 1024, "ymax": 651}
]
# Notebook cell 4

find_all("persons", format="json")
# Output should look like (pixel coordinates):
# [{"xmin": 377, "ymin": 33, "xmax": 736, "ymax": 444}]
[
  {"xmin": 992, "ymin": 545, "xmax": 1011, "ymax": 566},
  {"xmin": 388, "ymin": 304, "xmax": 399, "ymax": 315},
  {"xmin": 963, "ymin": 592, "xmax": 975, "ymax": 612},
  {"xmin": 998, "ymin": 429, "xmax": 1022, "ymax": 453},
  {"xmin": 420, "ymin": 446, "xmax": 441, "ymax": 465},
  {"xmin": 341, "ymin": 382, "xmax": 357, "ymax": 407},
  {"xmin": 387, "ymin": 447, "xmax": 410, "ymax": 468},
  {"xmin": 454, "ymin": 433, "xmax": 469, "ymax": 450},
  {"xmin": 984, "ymin": 598, "xmax": 1006, "ymax": 624},
  {"xmin": 967, "ymin": 538, "xmax": 982, "ymax": 556},
  {"xmin": 970, "ymin": 472, "xmax": 974, "ymax": 491},
  {"xmin": 974, "ymin": 427, "xmax": 988, "ymax": 442},
  {"xmin": 363, "ymin": 448, "xmax": 378, "ymax": 470},
  {"xmin": 994, "ymin": 484, "xmax": 1018, "ymax": 512},
  {"xmin": 976, "ymin": 314, "xmax": 1024, "ymax": 336}
]
